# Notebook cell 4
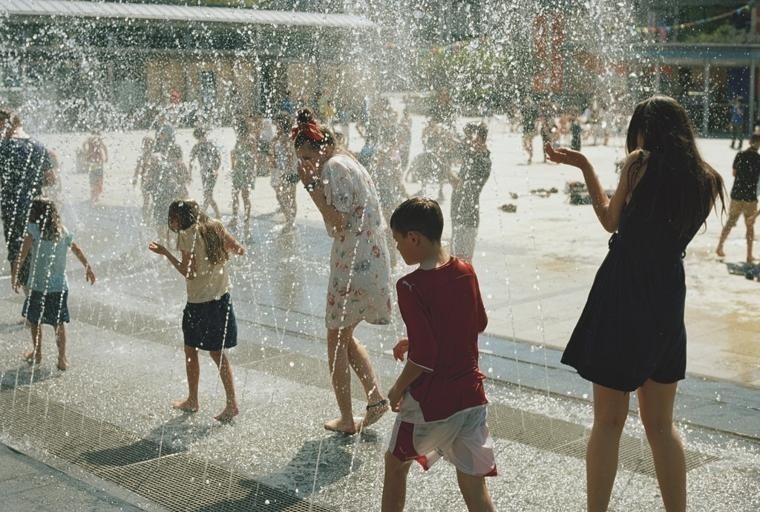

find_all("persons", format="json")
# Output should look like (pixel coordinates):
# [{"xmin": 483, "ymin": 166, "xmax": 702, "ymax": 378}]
[
  {"xmin": 12, "ymin": 197, "xmax": 98, "ymax": 372},
  {"xmin": 544, "ymin": 91, "xmax": 727, "ymax": 511},
  {"xmin": 283, "ymin": 82, "xmax": 492, "ymax": 263},
  {"xmin": 149, "ymin": 201, "xmax": 245, "ymax": 422},
  {"xmin": 731, "ymin": 94, "xmax": 750, "ymax": 148},
  {"xmin": 715, "ymin": 135, "xmax": 759, "ymax": 263},
  {"xmin": 79, "ymin": 106, "xmax": 301, "ymax": 235},
  {"xmin": 292, "ymin": 107, "xmax": 399, "ymax": 435},
  {"xmin": 503, "ymin": 91, "xmax": 609, "ymax": 166},
  {"xmin": 378, "ymin": 196, "xmax": 503, "ymax": 511},
  {"xmin": 0, "ymin": 109, "xmax": 57, "ymax": 294}
]
